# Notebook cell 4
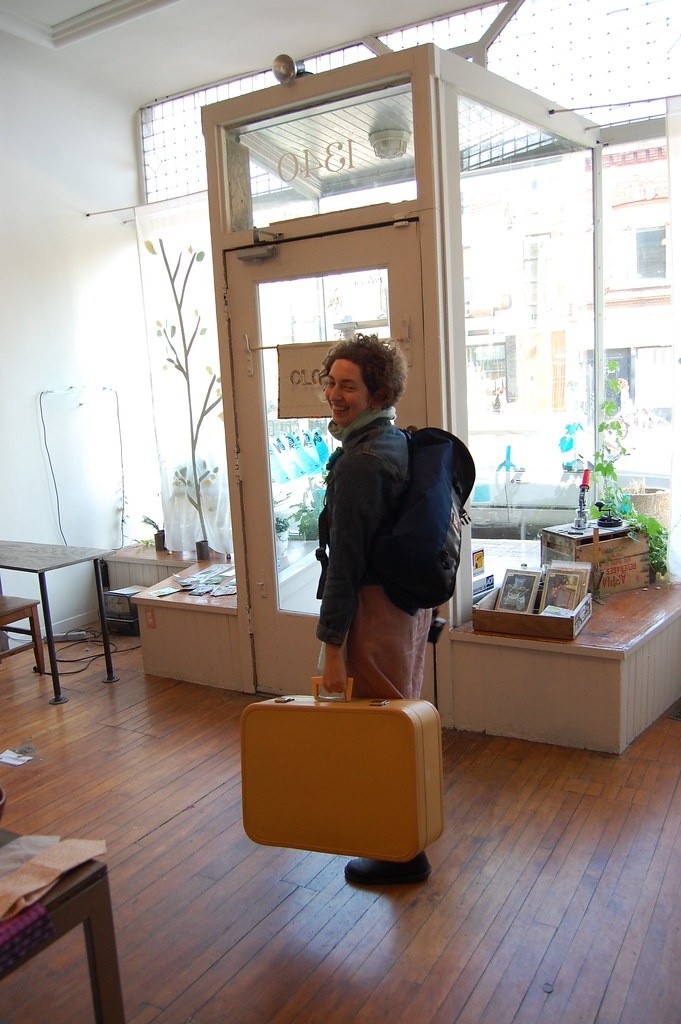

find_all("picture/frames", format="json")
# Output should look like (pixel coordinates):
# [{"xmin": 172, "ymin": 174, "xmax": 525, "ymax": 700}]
[
  {"xmin": 539, "ymin": 569, "xmax": 584, "ymax": 613},
  {"xmin": 549, "ymin": 567, "xmax": 589, "ymax": 603},
  {"xmin": 495, "ymin": 569, "xmax": 541, "ymax": 614}
]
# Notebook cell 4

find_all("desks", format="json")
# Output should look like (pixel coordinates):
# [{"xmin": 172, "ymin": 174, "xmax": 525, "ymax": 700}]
[{"xmin": 0, "ymin": 539, "xmax": 118, "ymax": 707}]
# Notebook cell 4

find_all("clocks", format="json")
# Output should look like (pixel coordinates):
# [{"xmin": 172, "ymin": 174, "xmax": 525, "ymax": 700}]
[{"xmin": 102, "ymin": 585, "xmax": 144, "ymax": 620}]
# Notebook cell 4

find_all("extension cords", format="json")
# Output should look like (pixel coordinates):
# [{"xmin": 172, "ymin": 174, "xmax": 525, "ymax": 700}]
[{"xmin": 44, "ymin": 631, "xmax": 87, "ymax": 644}]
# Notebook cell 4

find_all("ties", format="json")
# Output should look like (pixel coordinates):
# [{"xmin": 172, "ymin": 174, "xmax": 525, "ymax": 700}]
[{"xmin": 0, "ymin": 835, "xmax": 106, "ymax": 919}]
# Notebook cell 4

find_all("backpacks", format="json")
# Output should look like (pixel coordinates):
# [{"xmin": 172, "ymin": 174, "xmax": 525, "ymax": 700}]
[{"xmin": 316, "ymin": 429, "xmax": 475, "ymax": 615}]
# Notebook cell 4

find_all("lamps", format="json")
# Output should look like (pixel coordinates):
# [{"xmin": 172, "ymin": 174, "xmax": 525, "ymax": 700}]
[
  {"xmin": 272, "ymin": 53, "xmax": 305, "ymax": 84},
  {"xmin": 369, "ymin": 130, "xmax": 409, "ymax": 159}
]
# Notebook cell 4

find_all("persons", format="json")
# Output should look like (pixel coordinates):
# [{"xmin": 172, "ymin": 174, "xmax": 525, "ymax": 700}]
[
  {"xmin": 551, "ymin": 578, "xmax": 570, "ymax": 608},
  {"xmin": 314, "ymin": 332, "xmax": 433, "ymax": 886}
]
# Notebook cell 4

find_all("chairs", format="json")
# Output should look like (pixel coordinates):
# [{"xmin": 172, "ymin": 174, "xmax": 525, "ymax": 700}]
[
  {"xmin": 0, "ymin": 596, "xmax": 44, "ymax": 676},
  {"xmin": 0, "ymin": 829, "xmax": 124, "ymax": 1024}
]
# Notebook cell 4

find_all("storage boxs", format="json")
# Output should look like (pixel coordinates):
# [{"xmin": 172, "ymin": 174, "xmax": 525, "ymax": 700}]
[
  {"xmin": 472, "ymin": 585, "xmax": 593, "ymax": 640},
  {"xmin": 542, "ymin": 517, "xmax": 650, "ymax": 597}
]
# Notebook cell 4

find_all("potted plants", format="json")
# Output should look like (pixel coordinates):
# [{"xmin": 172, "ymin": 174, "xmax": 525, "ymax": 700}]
[
  {"xmin": 274, "ymin": 517, "xmax": 290, "ymax": 559},
  {"xmin": 619, "ymin": 479, "xmax": 670, "ymax": 534},
  {"xmin": 144, "ymin": 517, "xmax": 166, "ymax": 551}
]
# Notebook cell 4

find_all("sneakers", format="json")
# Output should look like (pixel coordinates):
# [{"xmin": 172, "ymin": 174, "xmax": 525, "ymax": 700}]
[{"xmin": 345, "ymin": 850, "xmax": 432, "ymax": 886}]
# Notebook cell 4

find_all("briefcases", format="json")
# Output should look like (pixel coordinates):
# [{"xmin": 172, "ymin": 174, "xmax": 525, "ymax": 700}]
[{"xmin": 240, "ymin": 676, "xmax": 444, "ymax": 862}]
